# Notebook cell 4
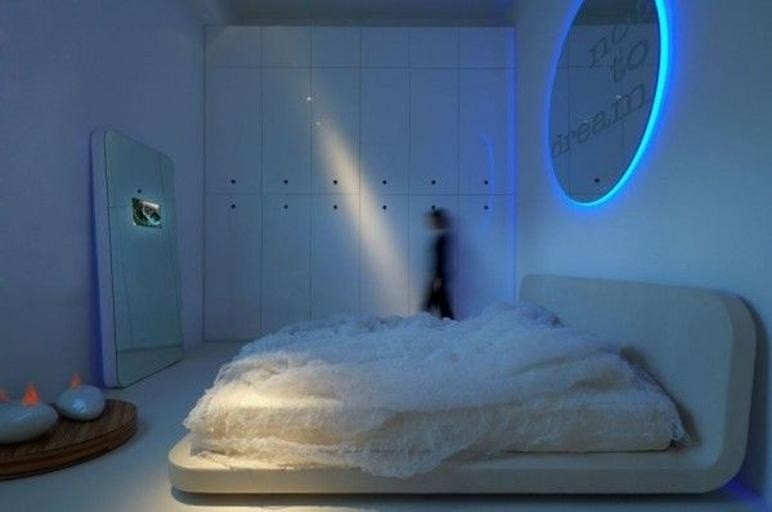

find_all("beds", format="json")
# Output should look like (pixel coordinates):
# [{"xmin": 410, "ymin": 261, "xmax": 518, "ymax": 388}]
[{"xmin": 169, "ymin": 273, "xmax": 757, "ymax": 499}]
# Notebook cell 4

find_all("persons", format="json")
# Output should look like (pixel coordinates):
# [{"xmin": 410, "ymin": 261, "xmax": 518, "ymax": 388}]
[{"xmin": 418, "ymin": 206, "xmax": 456, "ymax": 320}]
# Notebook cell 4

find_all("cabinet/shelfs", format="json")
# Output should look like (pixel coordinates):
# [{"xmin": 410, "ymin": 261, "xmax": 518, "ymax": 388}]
[{"xmin": 203, "ymin": 25, "xmax": 513, "ymax": 345}]
